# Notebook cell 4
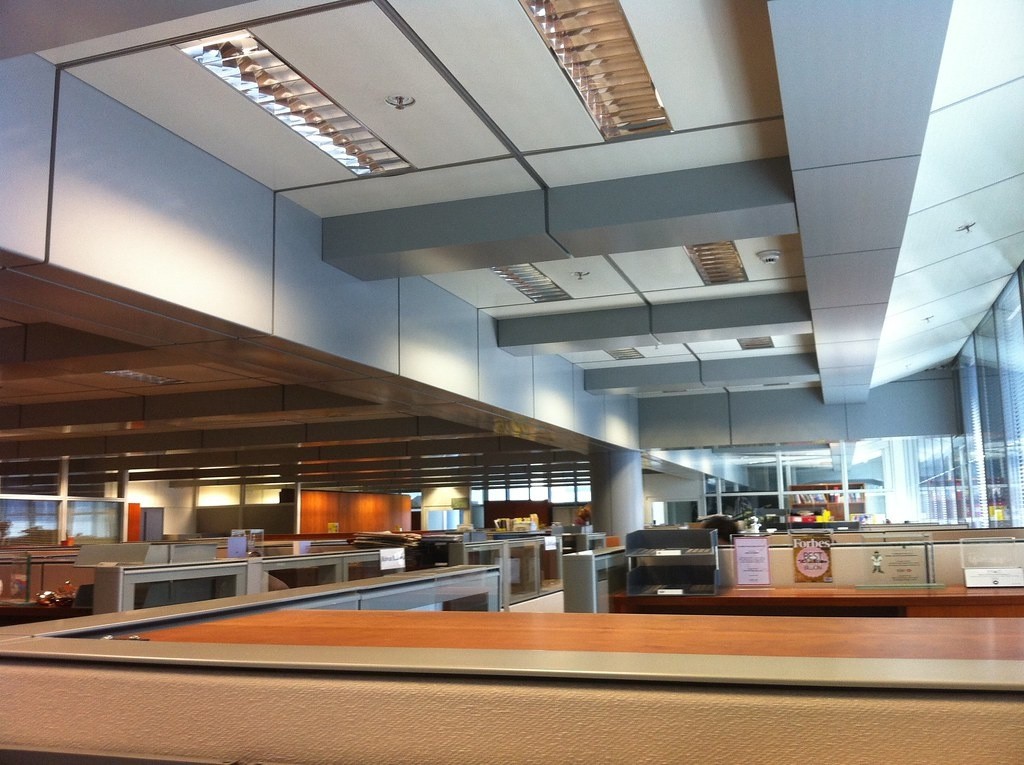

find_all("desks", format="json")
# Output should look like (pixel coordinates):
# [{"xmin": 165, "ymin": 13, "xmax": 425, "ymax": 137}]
[{"xmin": 0, "ymin": 528, "xmax": 1024, "ymax": 765}]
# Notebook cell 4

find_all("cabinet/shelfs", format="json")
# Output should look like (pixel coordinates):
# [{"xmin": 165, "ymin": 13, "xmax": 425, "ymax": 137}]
[
  {"xmin": 788, "ymin": 482, "xmax": 865, "ymax": 521},
  {"xmin": 623, "ymin": 528, "xmax": 719, "ymax": 596}
]
